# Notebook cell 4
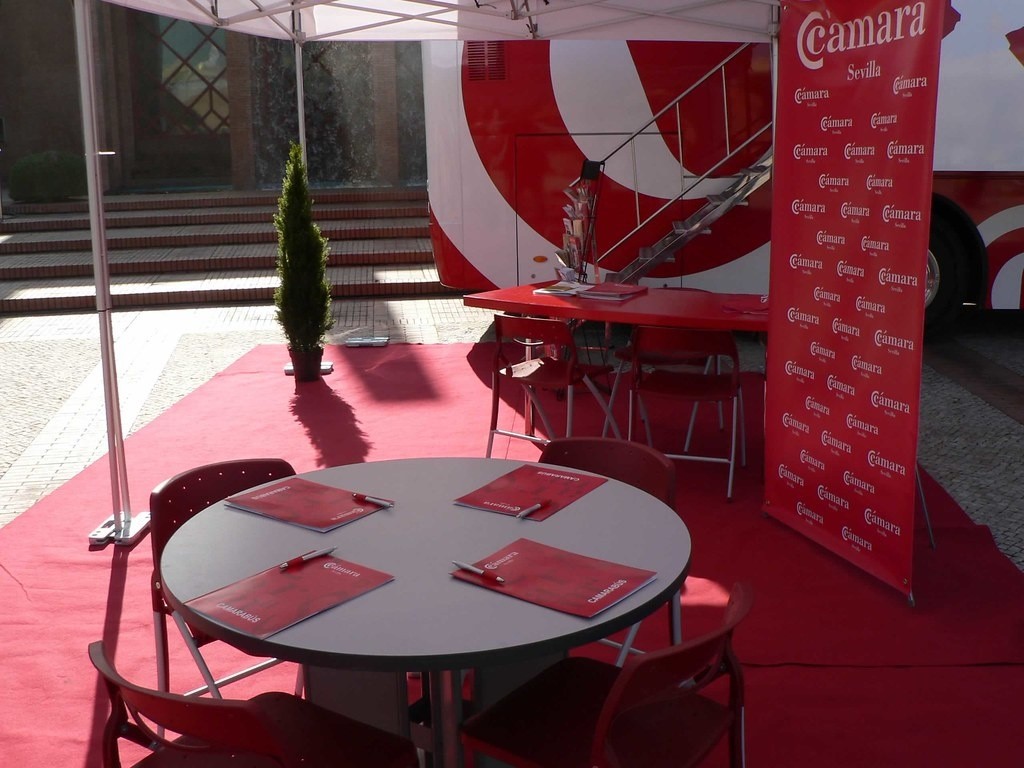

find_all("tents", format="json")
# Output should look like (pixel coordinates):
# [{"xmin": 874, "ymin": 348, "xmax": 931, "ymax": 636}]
[{"xmin": 74, "ymin": 0, "xmax": 781, "ymax": 532}]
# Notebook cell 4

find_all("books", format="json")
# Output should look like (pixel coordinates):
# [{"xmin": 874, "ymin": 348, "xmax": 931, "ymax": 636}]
[
  {"xmin": 533, "ymin": 281, "xmax": 595, "ymax": 295},
  {"xmin": 223, "ymin": 478, "xmax": 395, "ymax": 532},
  {"xmin": 554, "ymin": 185, "xmax": 590, "ymax": 281},
  {"xmin": 453, "ymin": 464, "xmax": 609, "ymax": 521},
  {"xmin": 577, "ymin": 282, "xmax": 648, "ymax": 300},
  {"xmin": 448, "ymin": 537, "xmax": 657, "ymax": 617},
  {"xmin": 182, "ymin": 549, "xmax": 394, "ymax": 639}
]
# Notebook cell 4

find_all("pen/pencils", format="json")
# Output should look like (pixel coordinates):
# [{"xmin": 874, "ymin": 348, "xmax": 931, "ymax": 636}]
[
  {"xmin": 279, "ymin": 546, "xmax": 337, "ymax": 569},
  {"xmin": 352, "ymin": 492, "xmax": 393, "ymax": 508},
  {"xmin": 515, "ymin": 498, "xmax": 552, "ymax": 518},
  {"xmin": 452, "ymin": 560, "xmax": 504, "ymax": 583}
]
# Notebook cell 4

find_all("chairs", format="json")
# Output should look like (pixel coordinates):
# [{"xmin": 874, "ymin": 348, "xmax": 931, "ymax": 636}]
[
  {"xmin": 87, "ymin": 641, "xmax": 422, "ymax": 768},
  {"xmin": 457, "ymin": 288, "xmax": 747, "ymax": 768},
  {"xmin": 149, "ymin": 459, "xmax": 308, "ymax": 740}
]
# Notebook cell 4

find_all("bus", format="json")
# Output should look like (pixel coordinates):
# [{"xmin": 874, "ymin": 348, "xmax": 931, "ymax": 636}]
[{"xmin": 421, "ymin": 0, "xmax": 1024, "ymax": 350}]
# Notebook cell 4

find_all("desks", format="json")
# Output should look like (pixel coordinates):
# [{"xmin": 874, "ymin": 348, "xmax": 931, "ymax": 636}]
[
  {"xmin": 174, "ymin": 457, "xmax": 692, "ymax": 768},
  {"xmin": 459, "ymin": 280, "xmax": 768, "ymax": 497}
]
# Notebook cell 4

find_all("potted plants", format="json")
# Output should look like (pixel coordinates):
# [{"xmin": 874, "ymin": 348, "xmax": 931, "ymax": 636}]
[{"xmin": 268, "ymin": 139, "xmax": 331, "ymax": 381}]
